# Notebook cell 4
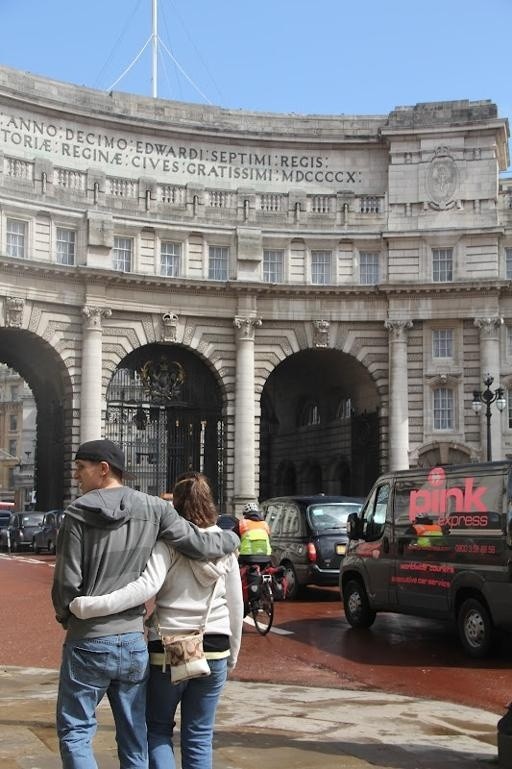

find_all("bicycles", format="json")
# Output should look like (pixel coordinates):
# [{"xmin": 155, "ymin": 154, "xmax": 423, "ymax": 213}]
[{"xmin": 243, "ymin": 564, "xmax": 275, "ymax": 636}]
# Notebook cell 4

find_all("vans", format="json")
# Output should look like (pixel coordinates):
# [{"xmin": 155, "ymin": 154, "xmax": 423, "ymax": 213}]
[{"xmin": 339, "ymin": 462, "xmax": 512, "ymax": 659}]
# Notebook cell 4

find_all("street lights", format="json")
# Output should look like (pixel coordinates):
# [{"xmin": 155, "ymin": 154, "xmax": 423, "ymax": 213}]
[{"xmin": 472, "ymin": 372, "xmax": 505, "ymax": 462}]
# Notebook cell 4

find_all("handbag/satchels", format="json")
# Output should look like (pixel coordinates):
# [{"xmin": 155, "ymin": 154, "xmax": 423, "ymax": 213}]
[{"xmin": 161, "ymin": 627, "xmax": 212, "ymax": 686}]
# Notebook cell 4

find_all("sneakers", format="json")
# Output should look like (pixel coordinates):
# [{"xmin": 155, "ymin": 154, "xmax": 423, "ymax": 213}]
[{"xmin": 244, "ymin": 601, "xmax": 250, "ymax": 618}]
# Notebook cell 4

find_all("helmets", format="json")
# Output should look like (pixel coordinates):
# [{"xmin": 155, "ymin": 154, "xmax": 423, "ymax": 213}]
[{"xmin": 242, "ymin": 502, "xmax": 259, "ymax": 515}]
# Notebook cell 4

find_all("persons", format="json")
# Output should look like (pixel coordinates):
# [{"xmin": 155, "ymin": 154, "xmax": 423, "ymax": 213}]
[
  {"xmin": 69, "ymin": 473, "xmax": 246, "ymax": 769},
  {"xmin": 44, "ymin": 438, "xmax": 242, "ymax": 768},
  {"xmin": 235, "ymin": 501, "xmax": 271, "ymax": 617}
]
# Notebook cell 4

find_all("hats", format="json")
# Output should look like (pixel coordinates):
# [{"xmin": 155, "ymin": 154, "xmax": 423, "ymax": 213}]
[{"xmin": 75, "ymin": 440, "xmax": 137, "ymax": 481}]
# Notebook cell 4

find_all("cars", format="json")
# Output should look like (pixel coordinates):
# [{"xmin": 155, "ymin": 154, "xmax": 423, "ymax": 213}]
[
  {"xmin": 0, "ymin": 509, "xmax": 68, "ymax": 554},
  {"xmin": 260, "ymin": 495, "xmax": 366, "ymax": 600}
]
